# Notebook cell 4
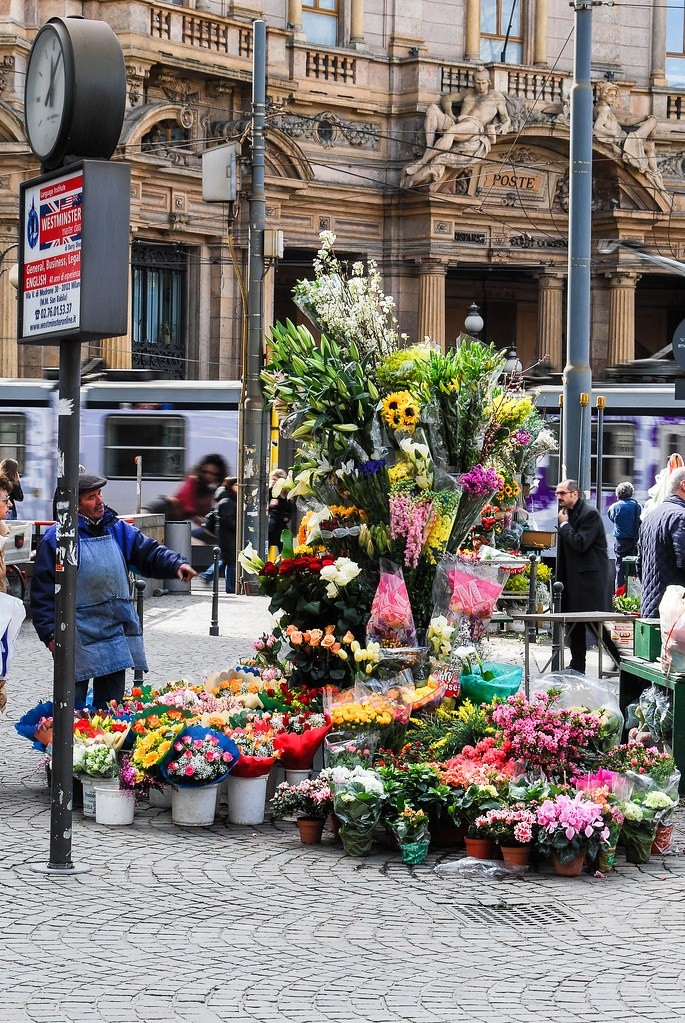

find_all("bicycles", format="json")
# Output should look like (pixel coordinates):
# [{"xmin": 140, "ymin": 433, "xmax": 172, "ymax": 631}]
[{"xmin": 4, "ymin": 563, "xmax": 28, "ymax": 601}]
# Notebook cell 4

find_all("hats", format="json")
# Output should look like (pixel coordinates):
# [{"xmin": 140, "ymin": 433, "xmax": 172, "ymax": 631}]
[{"xmin": 78, "ymin": 472, "xmax": 108, "ymax": 494}]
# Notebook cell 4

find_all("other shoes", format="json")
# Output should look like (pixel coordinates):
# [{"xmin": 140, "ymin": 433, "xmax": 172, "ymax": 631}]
[{"xmin": 558, "ymin": 667, "xmax": 585, "ymax": 677}]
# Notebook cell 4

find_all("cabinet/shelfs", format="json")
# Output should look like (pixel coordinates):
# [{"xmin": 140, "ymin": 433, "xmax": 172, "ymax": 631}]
[{"xmin": 617, "ymin": 655, "xmax": 685, "ymax": 795}]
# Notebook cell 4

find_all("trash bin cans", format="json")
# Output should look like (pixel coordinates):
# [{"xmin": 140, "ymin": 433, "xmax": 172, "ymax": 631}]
[
  {"xmin": 164, "ymin": 520, "xmax": 191, "ymax": 595},
  {"xmin": 622, "ymin": 555, "xmax": 639, "ymax": 586}
]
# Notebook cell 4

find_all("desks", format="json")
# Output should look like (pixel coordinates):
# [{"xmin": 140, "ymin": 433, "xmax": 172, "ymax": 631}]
[{"xmin": 508, "ymin": 608, "xmax": 644, "ymax": 679}]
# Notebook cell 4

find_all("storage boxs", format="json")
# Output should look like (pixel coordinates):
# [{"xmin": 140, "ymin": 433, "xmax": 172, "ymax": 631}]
[
  {"xmin": 602, "ymin": 618, "xmax": 634, "ymax": 657},
  {"xmin": 2, "ymin": 520, "xmax": 33, "ymax": 566},
  {"xmin": 635, "ymin": 618, "xmax": 662, "ymax": 663}
]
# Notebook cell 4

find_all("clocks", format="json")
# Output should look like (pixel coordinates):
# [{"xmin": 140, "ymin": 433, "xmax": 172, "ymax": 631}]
[{"xmin": 23, "ymin": 14, "xmax": 126, "ymax": 171}]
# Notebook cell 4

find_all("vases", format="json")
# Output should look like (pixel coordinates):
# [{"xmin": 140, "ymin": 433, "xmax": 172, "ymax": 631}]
[
  {"xmin": 401, "ymin": 838, "xmax": 430, "ymax": 866},
  {"xmin": 328, "ymin": 809, "xmax": 343, "ymax": 833},
  {"xmin": 502, "ymin": 604, "xmax": 528, "ymax": 634},
  {"xmin": 297, "ymin": 818, "xmax": 326, "ymax": 844},
  {"xmin": 45, "ymin": 762, "xmax": 311, "ymax": 827},
  {"xmin": 663, "ymin": 769, "xmax": 681, "ymax": 802},
  {"xmin": 463, "ymin": 824, "xmax": 673, "ymax": 880},
  {"xmin": 339, "ymin": 828, "xmax": 373, "ymax": 858}
]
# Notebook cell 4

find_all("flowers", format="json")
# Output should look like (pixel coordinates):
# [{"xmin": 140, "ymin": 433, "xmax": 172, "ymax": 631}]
[
  {"xmin": 14, "ymin": 671, "xmax": 329, "ymax": 786},
  {"xmin": 612, "ymin": 581, "xmax": 642, "ymax": 615},
  {"xmin": 329, "ymin": 675, "xmax": 676, "ymax": 843},
  {"xmin": 233, "ymin": 221, "xmax": 560, "ymax": 671},
  {"xmin": 270, "ymin": 777, "xmax": 334, "ymax": 819}
]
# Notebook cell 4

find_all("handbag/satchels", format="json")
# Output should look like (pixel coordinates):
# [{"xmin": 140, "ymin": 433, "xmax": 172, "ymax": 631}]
[{"xmin": 658, "ymin": 584, "xmax": 685, "ymax": 673}]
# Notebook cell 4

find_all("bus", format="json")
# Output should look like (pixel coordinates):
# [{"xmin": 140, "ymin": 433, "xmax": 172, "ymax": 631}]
[{"xmin": 0, "ymin": 358, "xmax": 685, "ymax": 568}]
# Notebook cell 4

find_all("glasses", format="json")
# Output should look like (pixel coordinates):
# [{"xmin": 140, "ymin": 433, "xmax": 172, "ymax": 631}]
[
  {"xmin": 201, "ymin": 469, "xmax": 219, "ymax": 477},
  {"xmin": 554, "ymin": 490, "xmax": 573, "ymax": 496},
  {"xmin": 1, "ymin": 496, "xmax": 11, "ymax": 503}
]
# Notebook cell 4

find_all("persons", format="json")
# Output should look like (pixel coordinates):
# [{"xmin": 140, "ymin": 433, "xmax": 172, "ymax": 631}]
[
  {"xmin": 200, "ymin": 469, "xmax": 293, "ymax": 592},
  {"xmin": 593, "ymin": 82, "xmax": 663, "ymax": 180},
  {"xmin": 607, "ymin": 482, "xmax": 642, "ymax": 589},
  {"xmin": 404, "ymin": 67, "xmax": 510, "ymax": 174},
  {"xmin": 0, "ymin": 458, "xmax": 23, "ymax": 709},
  {"xmin": 636, "ymin": 467, "xmax": 685, "ymax": 619},
  {"xmin": 140, "ymin": 453, "xmax": 228, "ymax": 527},
  {"xmin": 29, "ymin": 474, "xmax": 200, "ymax": 708},
  {"xmin": 556, "ymin": 479, "xmax": 626, "ymax": 675}
]
